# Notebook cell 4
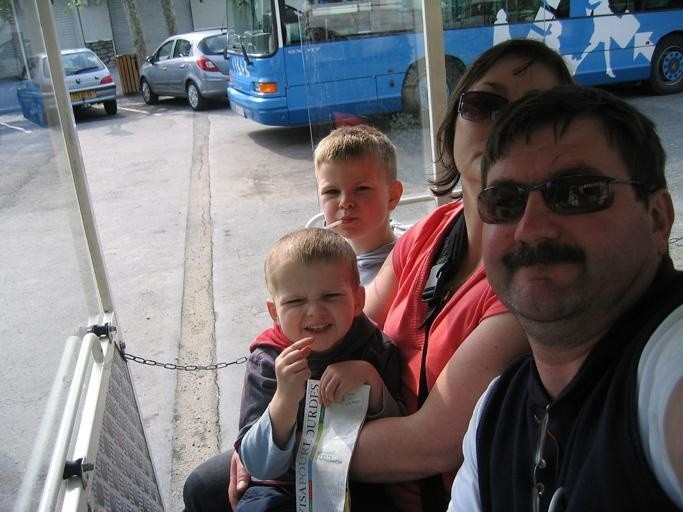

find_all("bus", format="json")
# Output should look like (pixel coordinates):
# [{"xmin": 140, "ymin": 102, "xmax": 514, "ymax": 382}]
[{"xmin": 225, "ymin": 2, "xmax": 682, "ymax": 125}]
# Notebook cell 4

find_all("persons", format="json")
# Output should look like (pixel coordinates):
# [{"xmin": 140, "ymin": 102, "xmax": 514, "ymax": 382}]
[
  {"xmin": 313, "ymin": 124, "xmax": 408, "ymax": 290},
  {"xmin": 182, "ymin": 39, "xmax": 571, "ymax": 511},
  {"xmin": 235, "ymin": 227, "xmax": 408, "ymax": 512},
  {"xmin": 443, "ymin": 84, "xmax": 683, "ymax": 512}
]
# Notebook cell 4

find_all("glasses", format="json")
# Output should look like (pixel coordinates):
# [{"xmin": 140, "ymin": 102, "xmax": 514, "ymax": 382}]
[
  {"xmin": 531, "ymin": 405, "xmax": 561, "ymax": 512},
  {"xmin": 476, "ymin": 173, "xmax": 638, "ymax": 225},
  {"xmin": 456, "ymin": 90, "xmax": 512, "ymax": 128}
]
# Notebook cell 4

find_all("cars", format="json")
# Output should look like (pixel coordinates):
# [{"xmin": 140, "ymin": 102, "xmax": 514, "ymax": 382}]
[
  {"xmin": 136, "ymin": 29, "xmax": 229, "ymax": 111},
  {"xmin": 17, "ymin": 49, "xmax": 117, "ymax": 127}
]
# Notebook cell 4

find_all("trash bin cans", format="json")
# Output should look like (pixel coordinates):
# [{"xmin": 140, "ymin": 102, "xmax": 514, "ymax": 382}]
[{"xmin": 115, "ymin": 52, "xmax": 141, "ymax": 97}]
